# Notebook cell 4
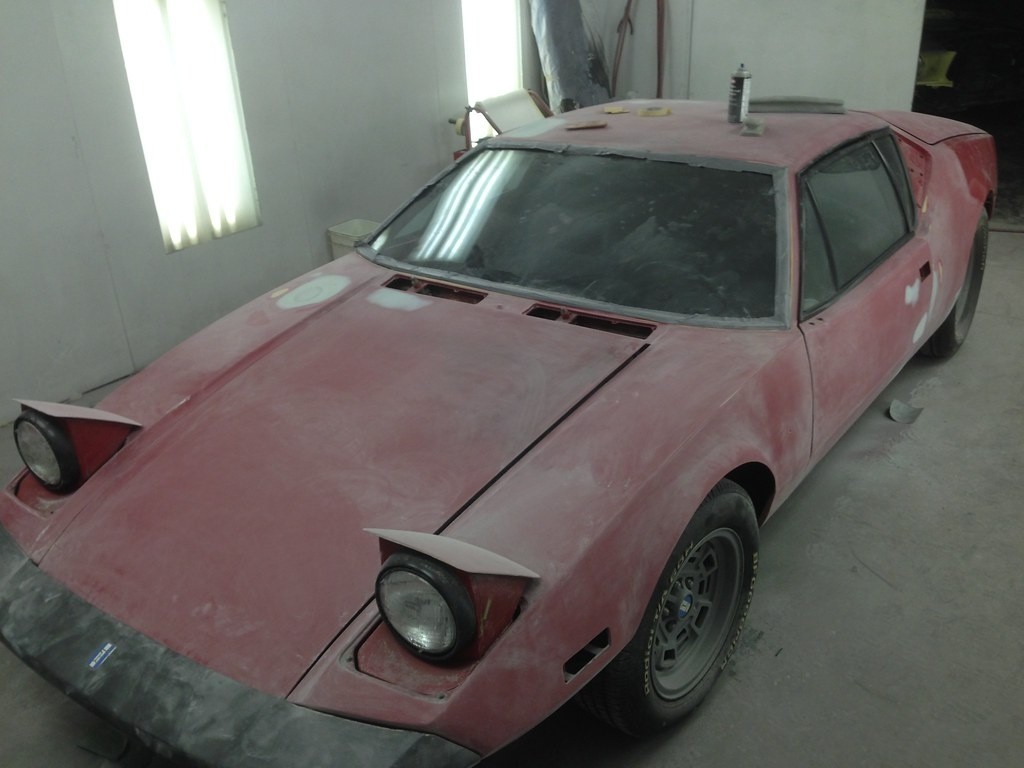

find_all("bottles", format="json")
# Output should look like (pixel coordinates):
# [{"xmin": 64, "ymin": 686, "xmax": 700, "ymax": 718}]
[{"xmin": 726, "ymin": 62, "xmax": 752, "ymax": 122}]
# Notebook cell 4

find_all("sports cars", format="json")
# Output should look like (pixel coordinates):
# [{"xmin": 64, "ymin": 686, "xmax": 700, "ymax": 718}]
[{"xmin": 0, "ymin": 96, "xmax": 996, "ymax": 766}]
